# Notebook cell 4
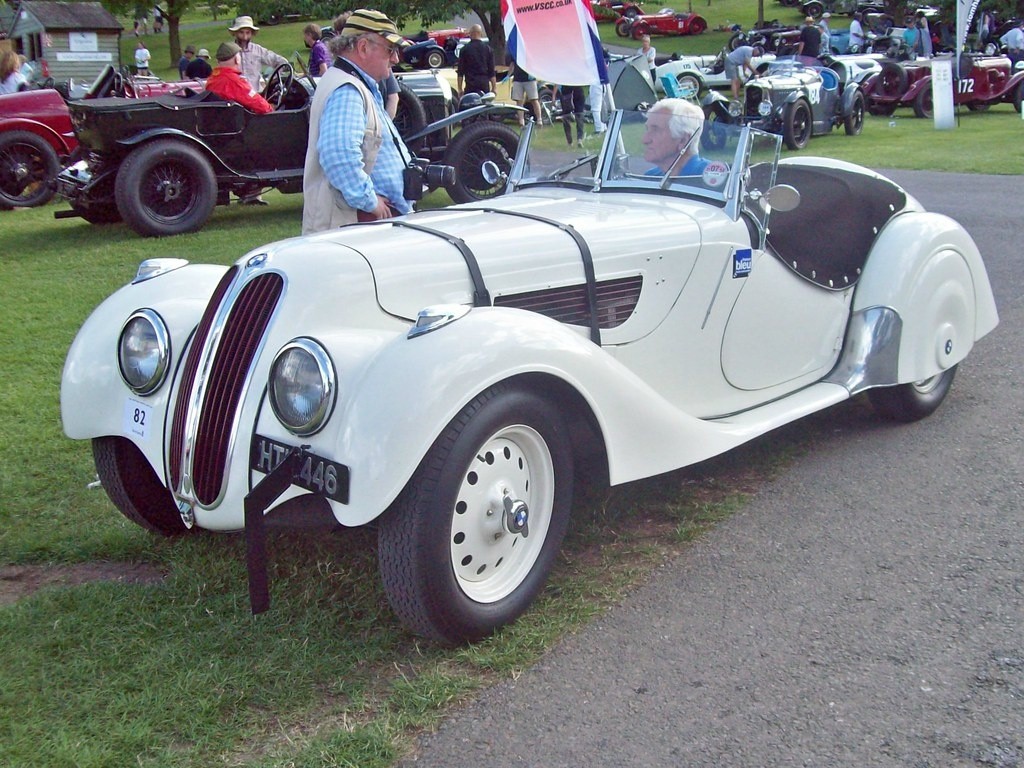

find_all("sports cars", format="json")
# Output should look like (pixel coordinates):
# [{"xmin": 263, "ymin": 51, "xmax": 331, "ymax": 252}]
[
  {"xmin": 1, "ymin": 0, "xmax": 1024, "ymax": 234},
  {"xmin": 59, "ymin": 106, "xmax": 1001, "ymax": 645}
]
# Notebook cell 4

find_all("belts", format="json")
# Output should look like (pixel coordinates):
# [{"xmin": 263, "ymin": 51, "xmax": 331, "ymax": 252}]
[{"xmin": 356, "ymin": 211, "xmax": 399, "ymax": 221}]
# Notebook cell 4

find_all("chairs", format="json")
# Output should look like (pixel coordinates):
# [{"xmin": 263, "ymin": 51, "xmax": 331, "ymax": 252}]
[
  {"xmin": 658, "ymin": 75, "xmax": 699, "ymax": 109},
  {"xmin": 665, "ymin": 72, "xmax": 698, "ymax": 105}
]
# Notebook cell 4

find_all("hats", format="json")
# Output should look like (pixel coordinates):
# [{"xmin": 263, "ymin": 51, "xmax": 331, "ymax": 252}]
[
  {"xmin": 196, "ymin": 49, "xmax": 211, "ymax": 60},
  {"xmin": 758, "ymin": 46, "xmax": 764, "ymax": 58},
  {"xmin": 805, "ymin": 17, "xmax": 815, "ymax": 22},
  {"xmin": 340, "ymin": 9, "xmax": 411, "ymax": 47},
  {"xmin": 228, "ymin": 16, "xmax": 260, "ymax": 36},
  {"xmin": 215, "ymin": 42, "xmax": 243, "ymax": 61},
  {"xmin": 822, "ymin": 13, "xmax": 831, "ymax": 18}
]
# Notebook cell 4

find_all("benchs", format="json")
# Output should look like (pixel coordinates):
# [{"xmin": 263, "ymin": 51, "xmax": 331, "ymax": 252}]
[
  {"xmin": 822, "ymin": 70, "xmax": 833, "ymax": 87},
  {"xmin": 746, "ymin": 161, "xmax": 877, "ymax": 282}
]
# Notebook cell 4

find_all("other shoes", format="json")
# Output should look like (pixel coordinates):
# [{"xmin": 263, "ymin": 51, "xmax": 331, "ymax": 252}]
[
  {"xmin": 569, "ymin": 143, "xmax": 573, "ymax": 148},
  {"xmin": 519, "ymin": 123, "xmax": 525, "ymax": 129},
  {"xmin": 578, "ymin": 142, "xmax": 583, "ymax": 148},
  {"xmin": 537, "ymin": 121, "xmax": 543, "ymax": 129}
]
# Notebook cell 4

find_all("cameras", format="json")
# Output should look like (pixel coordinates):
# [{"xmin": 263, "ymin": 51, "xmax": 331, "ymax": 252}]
[{"xmin": 403, "ymin": 157, "xmax": 456, "ymax": 200}]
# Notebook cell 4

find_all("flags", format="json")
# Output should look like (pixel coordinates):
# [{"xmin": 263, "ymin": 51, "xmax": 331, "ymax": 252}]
[
  {"xmin": 957, "ymin": 0, "xmax": 981, "ymax": 55},
  {"xmin": 501, "ymin": 0, "xmax": 609, "ymax": 87}
]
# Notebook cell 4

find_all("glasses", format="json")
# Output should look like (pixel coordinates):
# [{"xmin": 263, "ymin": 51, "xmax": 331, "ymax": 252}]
[{"xmin": 357, "ymin": 37, "xmax": 399, "ymax": 58}]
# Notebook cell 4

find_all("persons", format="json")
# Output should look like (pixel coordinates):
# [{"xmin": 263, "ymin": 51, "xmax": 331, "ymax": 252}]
[
  {"xmin": 228, "ymin": 16, "xmax": 289, "ymax": 92},
  {"xmin": 303, "ymin": 24, "xmax": 333, "ymax": 77},
  {"xmin": 642, "ymin": 98, "xmax": 710, "ymax": 176},
  {"xmin": 850, "ymin": 14, "xmax": 865, "ymax": 46},
  {"xmin": 725, "ymin": 46, "xmax": 764, "ymax": 98},
  {"xmin": 206, "ymin": 42, "xmax": 273, "ymax": 114},
  {"xmin": 553, "ymin": 51, "xmax": 607, "ymax": 148},
  {"xmin": 134, "ymin": 6, "xmax": 163, "ymax": 36},
  {"xmin": 0, "ymin": 51, "xmax": 33, "ymax": 95},
  {"xmin": 333, "ymin": 11, "xmax": 401, "ymax": 120},
  {"xmin": 903, "ymin": 12, "xmax": 932, "ymax": 59},
  {"xmin": 510, "ymin": 60, "xmax": 543, "ymax": 128},
  {"xmin": 136, "ymin": 41, "xmax": 151, "ymax": 76},
  {"xmin": 798, "ymin": 13, "xmax": 831, "ymax": 64},
  {"xmin": 1000, "ymin": 20, "xmax": 1024, "ymax": 63},
  {"xmin": 302, "ymin": 9, "xmax": 411, "ymax": 234},
  {"xmin": 637, "ymin": 37, "xmax": 656, "ymax": 84},
  {"xmin": 178, "ymin": 45, "xmax": 213, "ymax": 79},
  {"xmin": 458, "ymin": 24, "xmax": 498, "ymax": 98}
]
